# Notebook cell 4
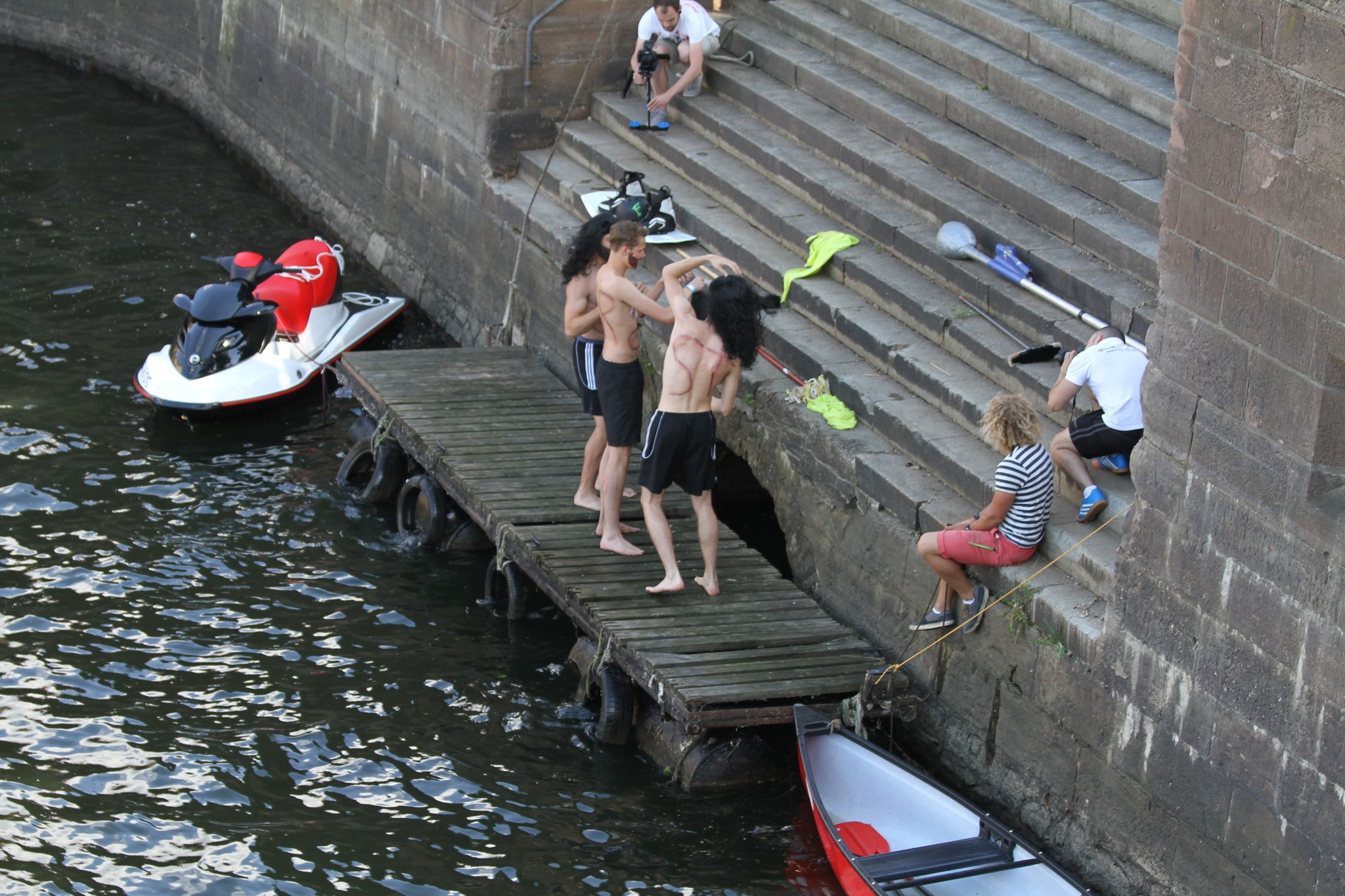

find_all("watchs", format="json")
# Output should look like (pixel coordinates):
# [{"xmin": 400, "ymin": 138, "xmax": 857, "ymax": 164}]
[{"xmin": 964, "ymin": 524, "xmax": 971, "ymax": 531}]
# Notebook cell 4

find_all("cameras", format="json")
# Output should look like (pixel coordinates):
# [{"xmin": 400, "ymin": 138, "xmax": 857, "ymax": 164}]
[{"xmin": 637, "ymin": 49, "xmax": 670, "ymax": 70}]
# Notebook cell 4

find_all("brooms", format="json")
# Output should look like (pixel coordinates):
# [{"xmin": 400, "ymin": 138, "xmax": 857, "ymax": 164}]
[
  {"xmin": 958, "ymin": 295, "xmax": 1064, "ymax": 366},
  {"xmin": 672, "ymin": 245, "xmax": 781, "ymax": 308}
]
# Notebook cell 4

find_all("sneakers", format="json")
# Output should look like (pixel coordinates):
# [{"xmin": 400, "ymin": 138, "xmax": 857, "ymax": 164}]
[
  {"xmin": 908, "ymin": 608, "xmax": 956, "ymax": 632},
  {"xmin": 635, "ymin": 184, "xmax": 676, "ymax": 235},
  {"xmin": 645, "ymin": 110, "xmax": 670, "ymax": 128},
  {"xmin": 1092, "ymin": 451, "xmax": 1129, "ymax": 474},
  {"xmin": 682, "ymin": 70, "xmax": 703, "ymax": 97},
  {"xmin": 960, "ymin": 585, "xmax": 989, "ymax": 634},
  {"xmin": 1074, "ymin": 487, "xmax": 1108, "ymax": 522},
  {"xmin": 598, "ymin": 170, "xmax": 644, "ymax": 217}
]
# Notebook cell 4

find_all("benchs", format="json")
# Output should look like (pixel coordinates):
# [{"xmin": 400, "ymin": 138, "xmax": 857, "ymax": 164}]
[{"xmin": 858, "ymin": 830, "xmax": 1015, "ymax": 882}]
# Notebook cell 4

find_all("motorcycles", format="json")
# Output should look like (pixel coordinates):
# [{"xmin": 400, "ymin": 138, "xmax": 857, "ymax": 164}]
[{"xmin": 132, "ymin": 237, "xmax": 408, "ymax": 419}]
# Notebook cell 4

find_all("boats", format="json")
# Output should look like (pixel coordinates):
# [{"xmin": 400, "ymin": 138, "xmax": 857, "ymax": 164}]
[{"xmin": 793, "ymin": 703, "xmax": 1100, "ymax": 896}]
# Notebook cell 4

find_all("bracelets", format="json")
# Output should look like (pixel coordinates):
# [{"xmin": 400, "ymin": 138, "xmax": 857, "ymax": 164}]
[
  {"xmin": 684, "ymin": 284, "xmax": 696, "ymax": 294},
  {"xmin": 973, "ymin": 511, "xmax": 980, "ymax": 520}
]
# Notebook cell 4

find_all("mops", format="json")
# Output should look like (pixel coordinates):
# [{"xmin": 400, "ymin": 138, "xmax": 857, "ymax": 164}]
[{"xmin": 753, "ymin": 345, "xmax": 836, "ymax": 405}]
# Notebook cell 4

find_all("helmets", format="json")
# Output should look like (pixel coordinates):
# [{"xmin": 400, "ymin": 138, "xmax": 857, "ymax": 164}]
[{"xmin": 615, "ymin": 195, "xmax": 651, "ymax": 223}]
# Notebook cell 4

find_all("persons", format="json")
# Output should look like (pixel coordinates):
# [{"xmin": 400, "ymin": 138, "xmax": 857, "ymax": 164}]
[
  {"xmin": 909, "ymin": 396, "xmax": 1053, "ymax": 636},
  {"xmin": 560, "ymin": 214, "xmax": 646, "ymax": 513},
  {"xmin": 637, "ymin": 254, "xmax": 763, "ymax": 597},
  {"xmin": 630, "ymin": 0, "xmax": 721, "ymax": 126},
  {"xmin": 1047, "ymin": 326, "xmax": 1149, "ymax": 523},
  {"xmin": 584, "ymin": 219, "xmax": 707, "ymax": 557}
]
